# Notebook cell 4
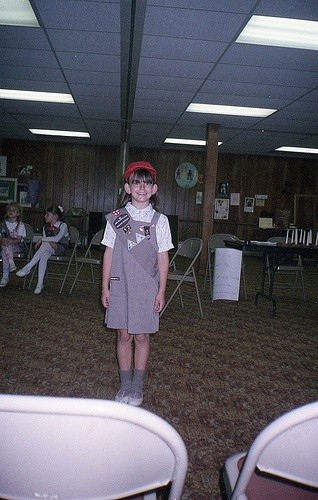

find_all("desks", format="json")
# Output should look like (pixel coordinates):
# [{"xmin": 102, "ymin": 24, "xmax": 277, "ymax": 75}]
[{"xmin": 223, "ymin": 240, "xmax": 318, "ymax": 317}]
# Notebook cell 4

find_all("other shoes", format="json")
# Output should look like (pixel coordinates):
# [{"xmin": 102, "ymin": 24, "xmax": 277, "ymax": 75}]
[
  {"xmin": 34, "ymin": 285, "xmax": 44, "ymax": 294},
  {"xmin": 115, "ymin": 394, "xmax": 130, "ymax": 404},
  {"xmin": 128, "ymin": 390, "xmax": 143, "ymax": 406},
  {"xmin": 16, "ymin": 269, "xmax": 30, "ymax": 277},
  {"xmin": 10, "ymin": 264, "xmax": 17, "ymax": 273},
  {"xmin": 0, "ymin": 278, "xmax": 9, "ymax": 287}
]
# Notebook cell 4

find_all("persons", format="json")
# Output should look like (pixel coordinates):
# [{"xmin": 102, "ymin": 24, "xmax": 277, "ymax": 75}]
[
  {"xmin": 102, "ymin": 161, "xmax": 174, "ymax": 407},
  {"xmin": 17, "ymin": 205, "xmax": 71, "ymax": 294},
  {"xmin": 0, "ymin": 202, "xmax": 27, "ymax": 287}
]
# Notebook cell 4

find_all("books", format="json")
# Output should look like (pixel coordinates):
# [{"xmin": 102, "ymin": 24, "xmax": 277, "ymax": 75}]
[{"xmin": 286, "ymin": 228, "xmax": 318, "ymax": 245}]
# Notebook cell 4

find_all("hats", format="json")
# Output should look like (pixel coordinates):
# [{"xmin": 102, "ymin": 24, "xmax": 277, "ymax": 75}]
[{"xmin": 123, "ymin": 161, "xmax": 156, "ymax": 184}]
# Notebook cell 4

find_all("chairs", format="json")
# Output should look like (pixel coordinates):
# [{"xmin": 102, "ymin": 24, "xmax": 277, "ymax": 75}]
[
  {"xmin": 159, "ymin": 237, "xmax": 203, "ymax": 318},
  {"xmin": 0, "ymin": 208, "xmax": 105, "ymax": 295},
  {"xmin": 223, "ymin": 401, "xmax": 318, "ymax": 500},
  {"xmin": 266, "ymin": 237, "xmax": 306, "ymax": 301},
  {"xmin": 0, "ymin": 394, "xmax": 188, "ymax": 500},
  {"xmin": 204, "ymin": 233, "xmax": 247, "ymax": 300}
]
